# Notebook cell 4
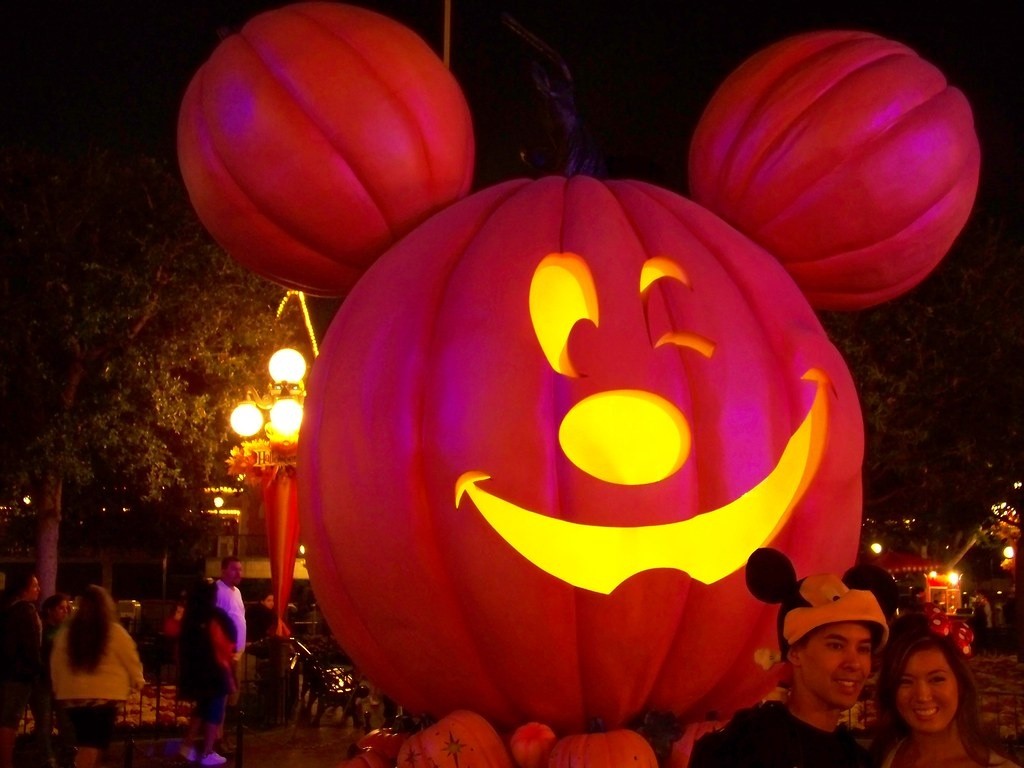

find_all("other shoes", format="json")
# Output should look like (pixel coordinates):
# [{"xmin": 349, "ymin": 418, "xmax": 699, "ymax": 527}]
[
  {"xmin": 200, "ymin": 752, "xmax": 227, "ymax": 766},
  {"xmin": 180, "ymin": 746, "xmax": 197, "ymax": 761}
]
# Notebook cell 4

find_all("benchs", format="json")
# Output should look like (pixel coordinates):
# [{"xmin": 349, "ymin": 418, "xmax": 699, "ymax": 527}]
[{"xmin": 285, "ymin": 635, "xmax": 366, "ymax": 729}]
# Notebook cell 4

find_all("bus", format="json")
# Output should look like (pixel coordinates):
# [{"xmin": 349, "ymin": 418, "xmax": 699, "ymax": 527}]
[{"xmin": 0, "ymin": 545, "xmax": 176, "ymax": 637}]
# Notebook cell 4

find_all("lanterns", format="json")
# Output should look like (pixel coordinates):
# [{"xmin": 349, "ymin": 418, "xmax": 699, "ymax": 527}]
[{"xmin": 340, "ymin": 709, "xmax": 661, "ymax": 768}]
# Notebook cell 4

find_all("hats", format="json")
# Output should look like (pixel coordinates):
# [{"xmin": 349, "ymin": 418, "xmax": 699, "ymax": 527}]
[{"xmin": 746, "ymin": 548, "xmax": 899, "ymax": 653}]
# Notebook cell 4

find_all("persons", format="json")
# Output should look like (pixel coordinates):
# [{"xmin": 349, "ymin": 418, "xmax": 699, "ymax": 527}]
[
  {"xmin": 0, "ymin": 553, "xmax": 298, "ymax": 768},
  {"xmin": 687, "ymin": 569, "xmax": 887, "ymax": 767},
  {"xmin": 873, "ymin": 613, "xmax": 1022, "ymax": 768}
]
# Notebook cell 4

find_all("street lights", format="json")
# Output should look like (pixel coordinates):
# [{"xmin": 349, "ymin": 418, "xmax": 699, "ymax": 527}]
[{"xmin": 229, "ymin": 348, "xmax": 311, "ymax": 637}]
